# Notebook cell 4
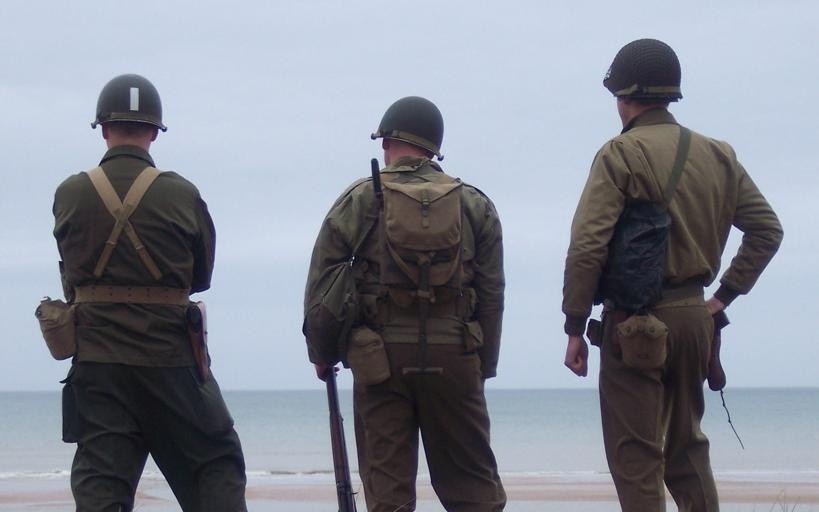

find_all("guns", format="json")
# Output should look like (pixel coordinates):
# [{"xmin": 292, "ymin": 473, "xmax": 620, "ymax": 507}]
[{"xmin": 326, "ymin": 366, "xmax": 357, "ymax": 512}]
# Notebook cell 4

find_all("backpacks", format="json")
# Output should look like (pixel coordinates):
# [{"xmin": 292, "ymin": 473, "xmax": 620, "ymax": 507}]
[{"xmin": 363, "ymin": 172, "xmax": 468, "ymax": 303}]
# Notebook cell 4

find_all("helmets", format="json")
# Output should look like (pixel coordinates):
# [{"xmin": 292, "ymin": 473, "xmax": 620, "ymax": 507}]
[
  {"xmin": 90, "ymin": 73, "xmax": 168, "ymax": 133},
  {"xmin": 602, "ymin": 39, "xmax": 684, "ymax": 100},
  {"xmin": 369, "ymin": 95, "xmax": 448, "ymax": 162}
]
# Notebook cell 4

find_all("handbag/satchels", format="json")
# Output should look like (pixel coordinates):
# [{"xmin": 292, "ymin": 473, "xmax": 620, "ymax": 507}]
[
  {"xmin": 304, "ymin": 259, "xmax": 360, "ymax": 368},
  {"xmin": 591, "ymin": 196, "xmax": 672, "ymax": 310}
]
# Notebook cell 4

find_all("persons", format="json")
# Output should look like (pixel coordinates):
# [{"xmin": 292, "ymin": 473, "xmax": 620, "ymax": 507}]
[
  {"xmin": 51, "ymin": 74, "xmax": 249, "ymax": 511},
  {"xmin": 301, "ymin": 95, "xmax": 507, "ymax": 512},
  {"xmin": 560, "ymin": 38, "xmax": 782, "ymax": 511}
]
